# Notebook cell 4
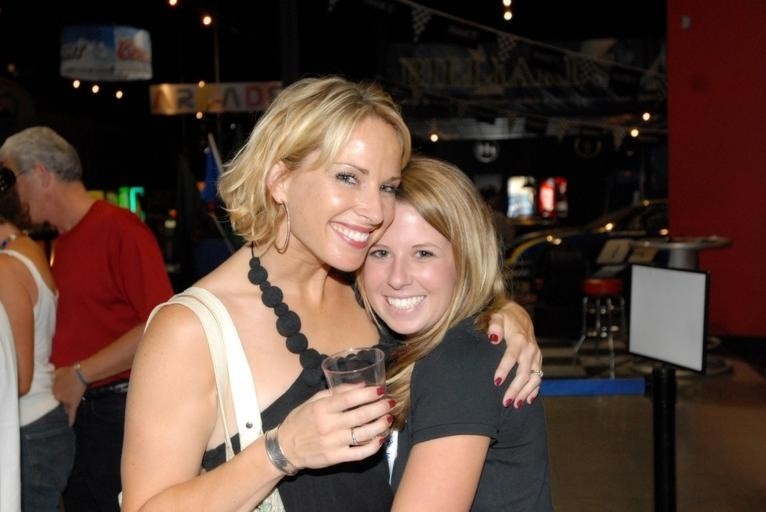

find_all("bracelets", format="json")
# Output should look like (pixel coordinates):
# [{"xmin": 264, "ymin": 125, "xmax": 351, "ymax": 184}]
[
  {"xmin": 74, "ymin": 363, "xmax": 91, "ymax": 386},
  {"xmin": 265, "ymin": 425, "xmax": 298, "ymax": 475}
]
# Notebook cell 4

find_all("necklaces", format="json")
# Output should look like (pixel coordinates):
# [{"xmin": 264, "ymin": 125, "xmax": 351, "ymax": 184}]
[
  {"xmin": 1, "ymin": 229, "xmax": 28, "ymax": 249},
  {"xmin": 246, "ymin": 235, "xmax": 399, "ymax": 387}
]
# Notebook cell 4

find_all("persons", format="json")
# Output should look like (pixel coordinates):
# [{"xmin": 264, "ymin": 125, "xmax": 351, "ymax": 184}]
[
  {"xmin": 0, "ymin": 181, "xmax": 77, "ymax": 512},
  {"xmin": 1, "ymin": 127, "xmax": 174, "ymax": 511},
  {"xmin": 357, "ymin": 157, "xmax": 553, "ymax": 512},
  {"xmin": 1, "ymin": 309, "xmax": 22, "ymax": 512},
  {"xmin": 120, "ymin": 75, "xmax": 545, "ymax": 512}
]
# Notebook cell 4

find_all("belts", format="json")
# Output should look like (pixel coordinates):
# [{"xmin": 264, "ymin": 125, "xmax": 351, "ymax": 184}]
[{"xmin": 82, "ymin": 382, "xmax": 128, "ymax": 401}]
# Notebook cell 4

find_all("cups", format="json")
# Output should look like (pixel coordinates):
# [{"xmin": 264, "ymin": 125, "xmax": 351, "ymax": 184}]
[{"xmin": 320, "ymin": 346, "xmax": 391, "ymax": 448}]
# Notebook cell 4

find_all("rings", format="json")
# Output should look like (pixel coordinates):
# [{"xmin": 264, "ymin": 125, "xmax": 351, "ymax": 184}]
[
  {"xmin": 532, "ymin": 369, "xmax": 544, "ymax": 377},
  {"xmin": 351, "ymin": 428, "xmax": 359, "ymax": 446}
]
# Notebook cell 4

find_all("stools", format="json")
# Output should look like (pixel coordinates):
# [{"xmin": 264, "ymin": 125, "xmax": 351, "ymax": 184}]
[{"xmin": 572, "ymin": 277, "xmax": 629, "ymax": 370}]
